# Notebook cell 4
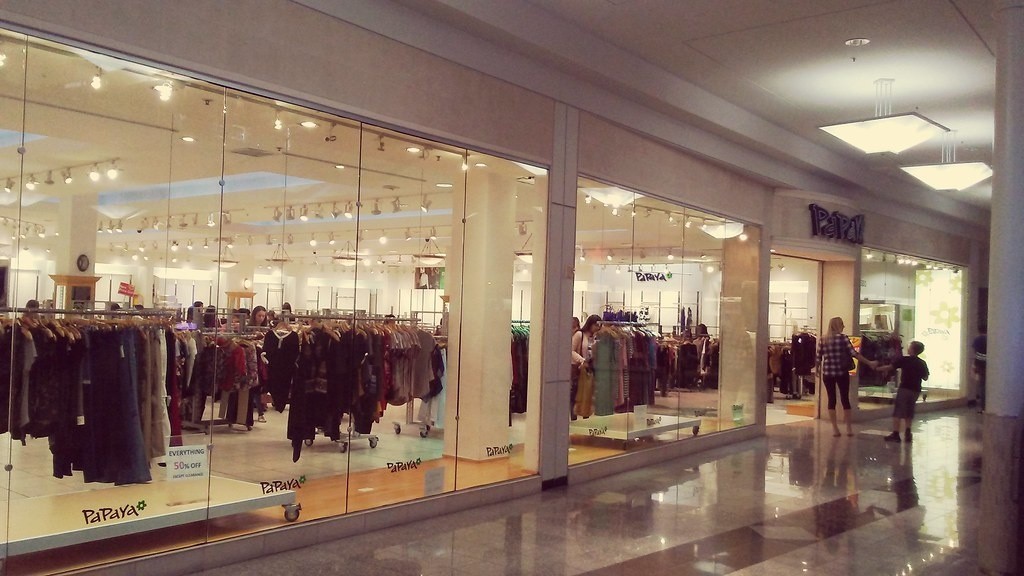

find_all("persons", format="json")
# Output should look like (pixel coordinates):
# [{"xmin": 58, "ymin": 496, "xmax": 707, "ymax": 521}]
[
  {"xmin": 573, "ymin": 317, "xmax": 580, "ymax": 332},
  {"xmin": 876, "ymin": 340, "xmax": 930, "ymax": 442},
  {"xmin": 383, "ymin": 314, "xmax": 396, "ymax": 325},
  {"xmin": 972, "ymin": 321, "xmax": 987, "ymax": 414},
  {"xmin": 697, "ymin": 323, "xmax": 710, "ymax": 339},
  {"xmin": 570, "ymin": 314, "xmax": 604, "ymax": 421},
  {"xmin": 110, "ymin": 302, "xmax": 151, "ymax": 320},
  {"xmin": 417, "ymin": 267, "xmax": 430, "ymax": 289},
  {"xmin": 238, "ymin": 305, "xmax": 273, "ymax": 422},
  {"xmin": 185, "ymin": 301, "xmax": 296, "ymax": 334},
  {"xmin": 814, "ymin": 316, "xmax": 878, "ymax": 437},
  {"xmin": 21, "ymin": 299, "xmax": 41, "ymax": 320}
]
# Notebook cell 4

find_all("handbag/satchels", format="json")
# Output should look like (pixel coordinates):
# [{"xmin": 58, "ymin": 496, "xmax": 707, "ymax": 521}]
[
  {"xmin": 570, "ymin": 364, "xmax": 579, "ymax": 382},
  {"xmin": 840, "ymin": 334, "xmax": 855, "ymax": 371}
]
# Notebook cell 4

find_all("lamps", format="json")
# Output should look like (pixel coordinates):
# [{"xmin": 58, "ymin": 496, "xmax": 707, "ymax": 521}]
[
  {"xmin": 26, "ymin": 174, "xmax": 35, "ymax": 190},
  {"xmin": 108, "ymin": 161, "xmax": 119, "ymax": 180},
  {"xmin": 10, "ymin": 195, "xmax": 445, "ymax": 274},
  {"xmin": 60, "ymin": 167, "xmax": 73, "ymax": 185},
  {"xmin": 86, "ymin": 163, "xmax": 102, "ymax": 182},
  {"xmin": 579, "ymin": 193, "xmax": 784, "ymax": 275},
  {"xmin": 4, "ymin": 178, "xmax": 16, "ymax": 192}
]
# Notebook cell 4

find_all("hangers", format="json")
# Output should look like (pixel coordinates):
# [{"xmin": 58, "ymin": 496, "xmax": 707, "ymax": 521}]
[
  {"xmin": 659, "ymin": 333, "xmax": 678, "ymax": 350},
  {"xmin": 861, "ymin": 330, "xmax": 905, "ymax": 344},
  {"xmin": 266, "ymin": 316, "xmax": 435, "ymax": 342},
  {"xmin": 594, "ymin": 321, "xmax": 662, "ymax": 340},
  {"xmin": 679, "ymin": 333, "xmax": 714, "ymax": 348},
  {"xmin": 0, "ymin": 304, "xmax": 184, "ymax": 341},
  {"xmin": 768, "ymin": 327, "xmax": 816, "ymax": 354},
  {"xmin": 511, "ymin": 319, "xmax": 530, "ymax": 342},
  {"xmin": 177, "ymin": 326, "xmax": 269, "ymax": 350}
]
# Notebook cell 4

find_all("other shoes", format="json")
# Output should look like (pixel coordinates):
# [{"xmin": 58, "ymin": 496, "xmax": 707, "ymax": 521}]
[
  {"xmin": 906, "ymin": 433, "xmax": 912, "ymax": 441},
  {"xmin": 884, "ymin": 434, "xmax": 901, "ymax": 442}
]
